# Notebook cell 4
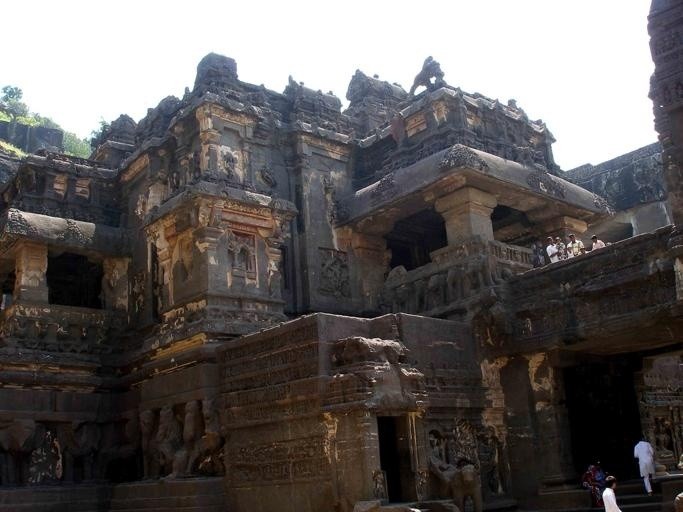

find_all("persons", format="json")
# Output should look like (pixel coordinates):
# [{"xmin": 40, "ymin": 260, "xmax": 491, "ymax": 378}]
[
  {"xmin": 545, "ymin": 236, "xmax": 560, "ymax": 265},
  {"xmin": 576, "ymin": 246, "xmax": 589, "ymax": 256},
  {"xmin": 566, "ymin": 233, "xmax": 583, "ymax": 257},
  {"xmin": 633, "ymin": 433, "xmax": 657, "ymax": 496},
  {"xmin": 581, "ymin": 458, "xmax": 608, "ymax": 508},
  {"xmin": 601, "ymin": 475, "xmax": 621, "ymax": 512},
  {"xmin": 564, "ymin": 247, "xmax": 577, "ymax": 259},
  {"xmin": 589, "ymin": 235, "xmax": 604, "ymax": 250},
  {"xmin": 554, "ymin": 236, "xmax": 566, "ymax": 261}
]
